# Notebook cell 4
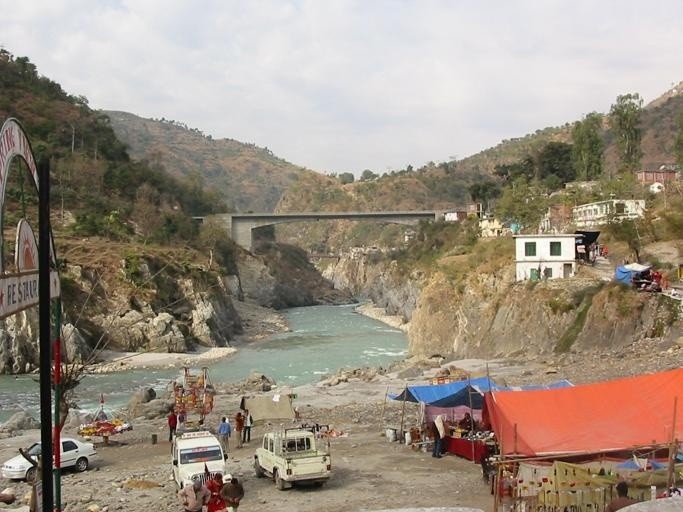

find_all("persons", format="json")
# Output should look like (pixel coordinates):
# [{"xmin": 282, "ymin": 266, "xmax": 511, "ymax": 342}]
[
  {"xmin": 649, "ymin": 270, "xmax": 669, "ymax": 292},
  {"xmin": 604, "ymin": 479, "xmax": 641, "ymax": 512},
  {"xmin": 166, "ymin": 403, "xmax": 253, "ymax": 511},
  {"xmin": 458, "ymin": 411, "xmax": 474, "ymax": 431},
  {"xmin": 431, "ymin": 412, "xmax": 446, "ymax": 461}
]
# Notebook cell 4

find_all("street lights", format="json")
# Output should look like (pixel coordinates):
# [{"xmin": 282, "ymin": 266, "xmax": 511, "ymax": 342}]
[{"xmin": 660, "ymin": 165, "xmax": 667, "ymax": 208}]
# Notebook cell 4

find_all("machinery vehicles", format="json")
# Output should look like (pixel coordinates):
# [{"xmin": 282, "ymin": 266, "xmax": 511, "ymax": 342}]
[{"xmin": 174, "ymin": 366, "xmax": 214, "ymax": 414}]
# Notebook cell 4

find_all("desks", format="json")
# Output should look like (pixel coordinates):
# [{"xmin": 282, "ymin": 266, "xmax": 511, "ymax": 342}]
[{"xmin": 443, "ymin": 435, "xmax": 496, "ymax": 464}]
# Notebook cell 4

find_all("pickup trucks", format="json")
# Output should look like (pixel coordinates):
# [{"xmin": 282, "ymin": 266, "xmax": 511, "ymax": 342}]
[{"xmin": 253, "ymin": 424, "xmax": 332, "ymax": 490}]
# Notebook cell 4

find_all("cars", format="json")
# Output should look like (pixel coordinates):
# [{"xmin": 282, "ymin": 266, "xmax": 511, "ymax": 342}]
[
  {"xmin": 2, "ymin": 438, "xmax": 99, "ymax": 483},
  {"xmin": 243, "ymin": 210, "xmax": 253, "ymax": 214}
]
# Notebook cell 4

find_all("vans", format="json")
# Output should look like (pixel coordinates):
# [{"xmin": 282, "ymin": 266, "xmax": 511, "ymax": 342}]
[{"xmin": 171, "ymin": 430, "xmax": 227, "ymax": 491}]
